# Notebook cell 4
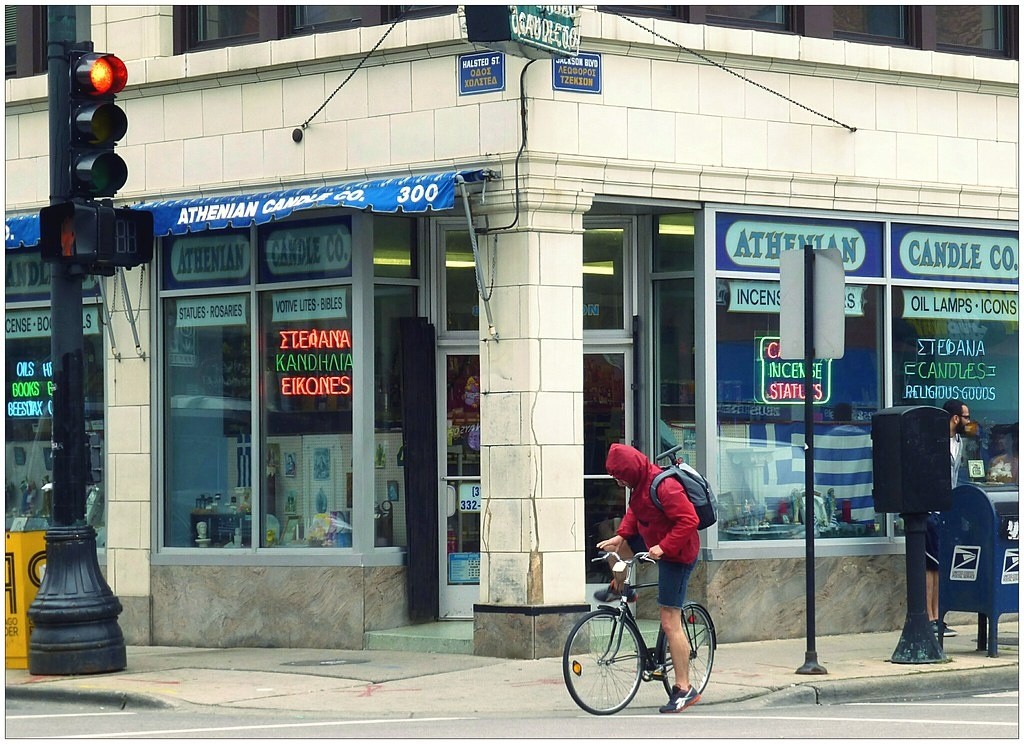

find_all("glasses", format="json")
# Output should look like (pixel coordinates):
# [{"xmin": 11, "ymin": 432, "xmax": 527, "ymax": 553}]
[{"xmin": 958, "ymin": 415, "xmax": 970, "ymax": 420}]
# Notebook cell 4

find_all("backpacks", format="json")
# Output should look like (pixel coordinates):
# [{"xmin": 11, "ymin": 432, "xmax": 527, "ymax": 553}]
[{"xmin": 650, "ymin": 463, "xmax": 718, "ymax": 530}]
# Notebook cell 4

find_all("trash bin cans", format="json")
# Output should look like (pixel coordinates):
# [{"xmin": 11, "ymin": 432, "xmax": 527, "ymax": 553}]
[{"xmin": 936, "ymin": 482, "xmax": 1019, "ymax": 657}]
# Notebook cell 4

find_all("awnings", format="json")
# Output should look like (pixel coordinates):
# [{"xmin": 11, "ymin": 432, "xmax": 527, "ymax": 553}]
[
  {"xmin": 121, "ymin": 168, "xmax": 502, "ymax": 359},
  {"xmin": 5, "ymin": 213, "xmax": 121, "ymax": 361}
]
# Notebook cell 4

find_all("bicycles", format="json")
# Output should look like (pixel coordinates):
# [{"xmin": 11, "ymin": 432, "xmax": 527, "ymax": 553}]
[{"xmin": 561, "ymin": 552, "xmax": 719, "ymax": 716}]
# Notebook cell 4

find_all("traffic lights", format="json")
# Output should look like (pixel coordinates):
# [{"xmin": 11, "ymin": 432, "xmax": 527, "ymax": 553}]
[
  {"xmin": 64, "ymin": 45, "xmax": 129, "ymax": 203},
  {"xmin": 39, "ymin": 204, "xmax": 104, "ymax": 270},
  {"xmin": 104, "ymin": 209, "xmax": 154, "ymax": 266}
]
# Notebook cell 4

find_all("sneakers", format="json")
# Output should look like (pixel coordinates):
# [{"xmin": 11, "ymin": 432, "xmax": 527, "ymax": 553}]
[
  {"xmin": 659, "ymin": 684, "xmax": 702, "ymax": 713},
  {"xmin": 930, "ymin": 619, "xmax": 958, "ymax": 637},
  {"xmin": 593, "ymin": 579, "xmax": 638, "ymax": 602}
]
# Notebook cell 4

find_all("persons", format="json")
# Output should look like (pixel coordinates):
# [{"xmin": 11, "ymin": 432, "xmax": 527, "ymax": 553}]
[
  {"xmin": 925, "ymin": 400, "xmax": 971, "ymax": 637},
  {"xmin": 987, "ymin": 422, "xmax": 1019, "ymax": 485},
  {"xmin": 596, "ymin": 444, "xmax": 701, "ymax": 713}
]
